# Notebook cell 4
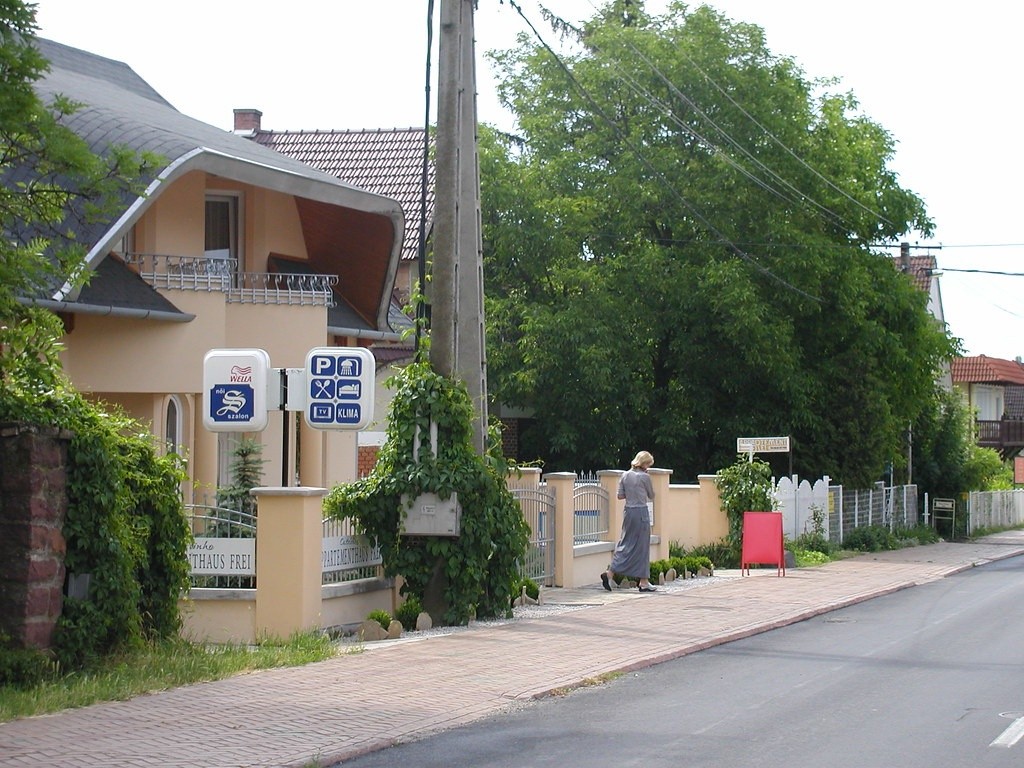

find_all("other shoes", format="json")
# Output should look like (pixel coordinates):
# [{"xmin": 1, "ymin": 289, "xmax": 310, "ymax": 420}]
[
  {"xmin": 639, "ymin": 582, "xmax": 657, "ymax": 592},
  {"xmin": 601, "ymin": 573, "xmax": 612, "ymax": 591}
]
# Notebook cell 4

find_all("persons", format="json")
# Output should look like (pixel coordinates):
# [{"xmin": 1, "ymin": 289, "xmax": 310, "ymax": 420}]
[{"xmin": 601, "ymin": 451, "xmax": 658, "ymax": 591}]
